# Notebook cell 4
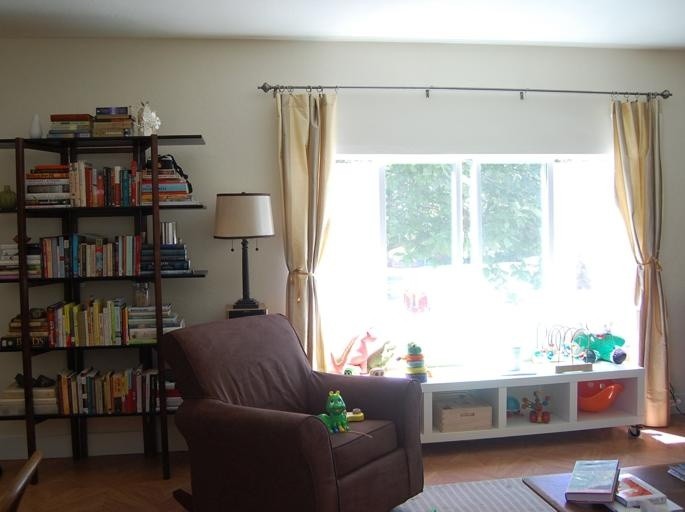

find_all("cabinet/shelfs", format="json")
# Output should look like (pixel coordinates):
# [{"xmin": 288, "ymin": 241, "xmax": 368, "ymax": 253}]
[
  {"xmin": 1, "ymin": 128, "xmax": 206, "ymax": 487},
  {"xmin": 383, "ymin": 362, "xmax": 646, "ymax": 448}
]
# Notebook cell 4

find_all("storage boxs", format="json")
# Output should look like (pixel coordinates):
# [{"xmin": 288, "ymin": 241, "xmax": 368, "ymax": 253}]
[{"xmin": 432, "ymin": 391, "xmax": 493, "ymax": 433}]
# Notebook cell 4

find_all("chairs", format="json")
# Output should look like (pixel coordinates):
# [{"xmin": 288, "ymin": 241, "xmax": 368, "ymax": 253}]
[{"xmin": 159, "ymin": 313, "xmax": 426, "ymax": 512}]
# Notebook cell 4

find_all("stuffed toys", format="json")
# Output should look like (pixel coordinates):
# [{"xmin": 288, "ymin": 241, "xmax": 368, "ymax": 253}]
[
  {"xmin": 575, "ymin": 317, "xmax": 626, "ymax": 363},
  {"xmin": 507, "ymin": 391, "xmax": 551, "ymax": 424}
]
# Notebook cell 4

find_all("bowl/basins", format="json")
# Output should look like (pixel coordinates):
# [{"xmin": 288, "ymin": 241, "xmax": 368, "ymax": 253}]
[{"xmin": 578, "ymin": 382, "xmax": 625, "ymax": 412}]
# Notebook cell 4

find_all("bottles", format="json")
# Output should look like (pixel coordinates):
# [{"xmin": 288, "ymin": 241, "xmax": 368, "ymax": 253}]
[
  {"xmin": 132, "ymin": 282, "xmax": 149, "ymax": 307},
  {"xmin": 0, "ymin": 185, "xmax": 16, "ymax": 210}
]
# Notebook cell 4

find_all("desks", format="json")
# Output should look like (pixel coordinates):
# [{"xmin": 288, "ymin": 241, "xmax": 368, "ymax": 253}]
[{"xmin": 521, "ymin": 463, "xmax": 685, "ymax": 512}]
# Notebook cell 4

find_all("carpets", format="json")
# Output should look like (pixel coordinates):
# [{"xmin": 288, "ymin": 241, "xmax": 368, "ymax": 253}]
[{"xmin": 392, "ymin": 473, "xmax": 556, "ymax": 512}]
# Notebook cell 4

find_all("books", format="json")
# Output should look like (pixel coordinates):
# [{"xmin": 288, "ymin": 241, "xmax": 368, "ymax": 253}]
[
  {"xmin": 25, "ymin": 160, "xmax": 202, "ymax": 209},
  {"xmin": 44, "ymin": 107, "xmax": 135, "ymax": 138},
  {"xmin": 0, "ymin": 297, "xmax": 184, "ymax": 415},
  {"xmin": 0, "ymin": 221, "xmax": 189, "ymax": 279},
  {"xmin": 433, "ymin": 397, "xmax": 492, "ymax": 432},
  {"xmin": 564, "ymin": 459, "xmax": 685, "ymax": 512}
]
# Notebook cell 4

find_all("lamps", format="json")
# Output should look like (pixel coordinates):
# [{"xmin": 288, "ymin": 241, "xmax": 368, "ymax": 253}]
[{"xmin": 211, "ymin": 189, "xmax": 277, "ymax": 321}]
